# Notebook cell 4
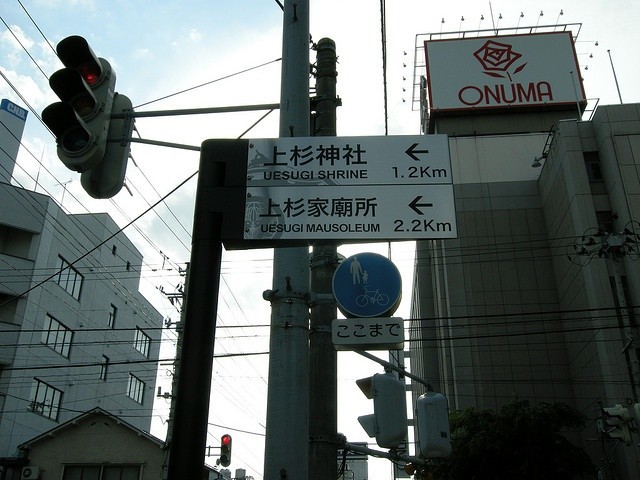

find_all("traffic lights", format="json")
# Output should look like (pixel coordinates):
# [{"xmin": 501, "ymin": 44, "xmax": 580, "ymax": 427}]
[
  {"xmin": 221, "ymin": 434, "xmax": 232, "ymax": 467},
  {"xmin": 602, "ymin": 403, "xmax": 631, "ymax": 445},
  {"xmin": 41, "ymin": 35, "xmax": 116, "ymax": 172},
  {"xmin": 355, "ymin": 373, "xmax": 407, "ymax": 448},
  {"xmin": 632, "ymin": 402, "xmax": 639, "ymax": 432},
  {"xmin": 416, "ymin": 391, "xmax": 451, "ymax": 459}
]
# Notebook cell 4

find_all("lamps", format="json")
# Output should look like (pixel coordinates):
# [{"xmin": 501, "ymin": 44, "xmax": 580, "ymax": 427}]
[{"xmin": 532, "ymin": 154, "xmax": 546, "ymax": 168}]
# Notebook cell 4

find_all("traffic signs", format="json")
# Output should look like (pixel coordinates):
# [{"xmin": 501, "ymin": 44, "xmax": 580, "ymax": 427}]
[
  {"xmin": 246, "ymin": 134, "xmax": 453, "ymax": 187},
  {"xmin": 243, "ymin": 184, "xmax": 458, "ymax": 239},
  {"xmin": 81, "ymin": 92, "xmax": 133, "ymax": 198},
  {"xmin": 332, "ymin": 252, "xmax": 402, "ymax": 317}
]
